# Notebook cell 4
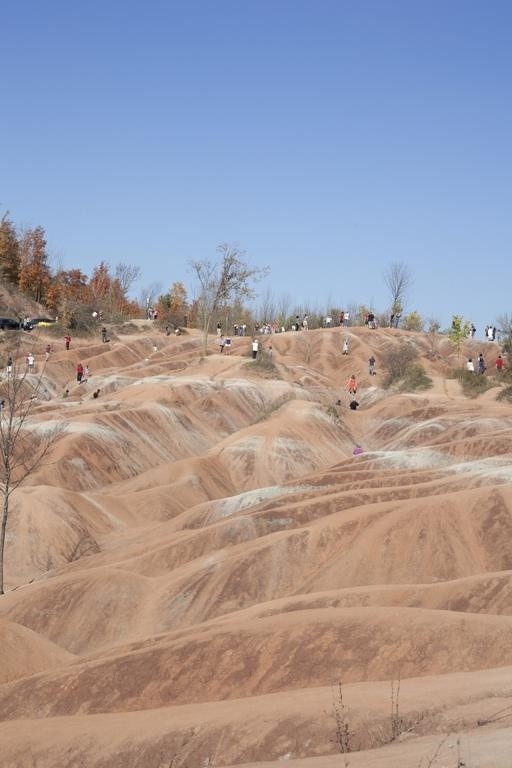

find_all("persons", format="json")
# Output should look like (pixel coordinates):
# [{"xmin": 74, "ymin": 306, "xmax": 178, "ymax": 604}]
[
  {"xmin": 451, "ymin": 322, "xmax": 503, "ymax": 343},
  {"xmin": 216, "ymin": 321, "xmax": 247, "ymax": 356},
  {"xmin": 466, "ymin": 353, "xmax": 505, "ymax": 377},
  {"xmin": 353, "ymin": 443, "xmax": 364, "ymax": 455},
  {"xmin": 8, "ymin": 310, "xmax": 108, "ymax": 400},
  {"xmin": 325, "ymin": 311, "xmax": 401, "ymax": 329},
  {"xmin": 335, "ymin": 338, "xmax": 375, "ymax": 410},
  {"xmin": 146, "ymin": 307, "xmax": 181, "ymax": 336},
  {"xmin": 252, "ymin": 314, "xmax": 309, "ymax": 369}
]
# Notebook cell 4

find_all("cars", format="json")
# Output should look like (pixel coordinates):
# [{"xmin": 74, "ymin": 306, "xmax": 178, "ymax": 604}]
[{"xmin": 0, "ymin": 316, "xmax": 57, "ymax": 332}]
